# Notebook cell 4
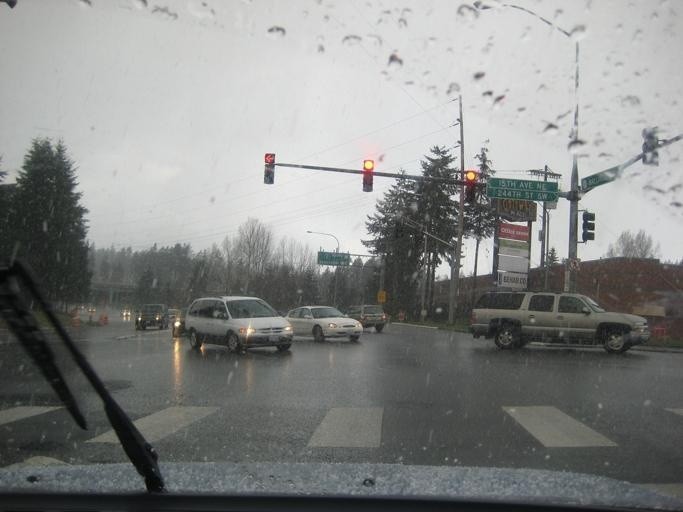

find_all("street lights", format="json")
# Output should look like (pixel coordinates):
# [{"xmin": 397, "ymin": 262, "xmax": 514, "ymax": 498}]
[
  {"xmin": 304, "ymin": 229, "xmax": 340, "ymax": 309},
  {"xmin": 473, "ymin": 1, "xmax": 582, "ymax": 300},
  {"xmin": 396, "ymin": 188, "xmax": 430, "ymax": 324}
]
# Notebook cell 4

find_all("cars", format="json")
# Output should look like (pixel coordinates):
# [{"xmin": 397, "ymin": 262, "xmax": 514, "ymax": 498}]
[
  {"xmin": 283, "ymin": 307, "xmax": 363, "ymax": 343},
  {"xmin": 172, "ymin": 308, "xmax": 188, "ymax": 337},
  {"xmin": 120, "ymin": 309, "xmax": 131, "ymax": 317},
  {"xmin": 167, "ymin": 309, "xmax": 181, "ymax": 322},
  {"xmin": 80, "ymin": 304, "xmax": 96, "ymax": 312}
]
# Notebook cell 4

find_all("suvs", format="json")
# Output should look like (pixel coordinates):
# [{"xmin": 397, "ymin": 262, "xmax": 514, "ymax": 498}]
[
  {"xmin": 134, "ymin": 304, "xmax": 169, "ymax": 330},
  {"xmin": 185, "ymin": 296, "xmax": 294, "ymax": 356},
  {"xmin": 348, "ymin": 304, "xmax": 386, "ymax": 332}
]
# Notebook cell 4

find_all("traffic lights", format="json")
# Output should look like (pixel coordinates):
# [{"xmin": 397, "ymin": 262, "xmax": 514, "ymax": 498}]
[
  {"xmin": 263, "ymin": 152, "xmax": 275, "ymax": 185},
  {"xmin": 581, "ymin": 210, "xmax": 594, "ymax": 241},
  {"xmin": 362, "ymin": 159, "xmax": 375, "ymax": 192},
  {"xmin": 641, "ymin": 124, "xmax": 659, "ymax": 167},
  {"xmin": 463, "ymin": 171, "xmax": 476, "ymax": 203}
]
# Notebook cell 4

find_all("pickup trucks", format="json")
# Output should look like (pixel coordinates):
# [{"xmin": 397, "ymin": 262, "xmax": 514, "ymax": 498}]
[{"xmin": 467, "ymin": 289, "xmax": 651, "ymax": 357}]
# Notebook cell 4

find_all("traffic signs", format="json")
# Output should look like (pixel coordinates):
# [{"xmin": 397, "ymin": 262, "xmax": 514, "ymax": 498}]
[
  {"xmin": 485, "ymin": 176, "xmax": 557, "ymax": 192},
  {"xmin": 485, "ymin": 186, "xmax": 559, "ymax": 204},
  {"xmin": 317, "ymin": 251, "xmax": 348, "ymax": 267},
  {"xmin": 581, "ymin": 169, "xmax": 613, "ymax": 191}
]
